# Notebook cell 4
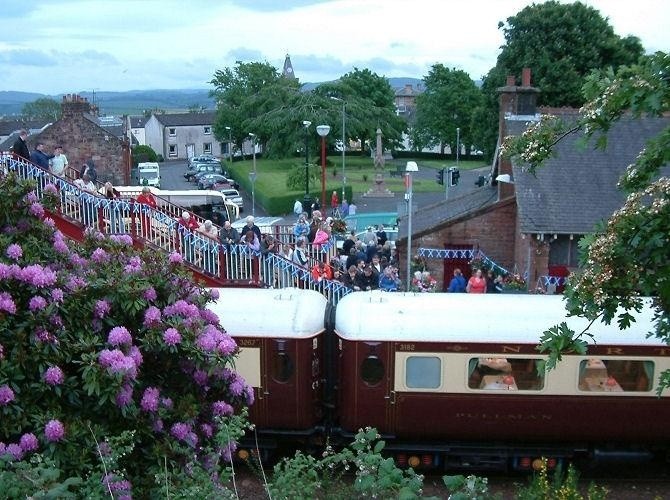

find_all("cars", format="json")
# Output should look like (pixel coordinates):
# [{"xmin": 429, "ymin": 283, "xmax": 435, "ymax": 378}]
[
  {"xmin": 336, "ymin": 227, "xmax": 399, "ymax": 252},
  {"xmin": 184, "ymin": 154, "xmax": 243, "ymax": 207}
]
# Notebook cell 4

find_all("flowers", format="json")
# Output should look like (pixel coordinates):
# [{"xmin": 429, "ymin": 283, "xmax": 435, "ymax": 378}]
[{"xmin": 0, "ymin": 182, "xmax": 256, "ymax": 499}]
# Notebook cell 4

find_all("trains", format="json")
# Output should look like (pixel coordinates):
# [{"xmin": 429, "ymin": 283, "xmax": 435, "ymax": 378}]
[{"xmin": 195, "ymin": 288, "xmax": 669, "ymax": 481}]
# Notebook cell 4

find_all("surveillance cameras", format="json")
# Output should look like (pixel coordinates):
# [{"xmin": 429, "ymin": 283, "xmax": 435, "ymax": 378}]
[{"xmin": 405, "ymin": 193, "xmax": 410, "ymax": 202}]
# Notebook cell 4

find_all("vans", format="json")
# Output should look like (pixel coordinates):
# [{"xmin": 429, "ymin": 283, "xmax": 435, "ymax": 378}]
[
  {"xmin": 342, "ymin": 211, "xmax": 399, "ymax": 237},
  {"xmin": 231, "ymin": 217, "xmax": 286, "ymax": 234}
]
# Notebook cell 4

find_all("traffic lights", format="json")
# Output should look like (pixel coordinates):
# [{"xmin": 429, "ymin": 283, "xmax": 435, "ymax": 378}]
[
  {"xmin": 452, "ymin": 170, "xmax": 460, "ymax": 186},
  {"xmin": 436, "ymin": 170, "xmax": 443, "ymax": 185}
]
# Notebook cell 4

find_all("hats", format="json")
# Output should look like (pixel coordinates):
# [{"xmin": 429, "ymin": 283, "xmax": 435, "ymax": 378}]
[
  {"xmin": 182, "ymin": 211, "xmax": 191, "ymax": 220},
  {"xmin": 299, "ymin": 214, "xmax": 304, "ymax": 222}
]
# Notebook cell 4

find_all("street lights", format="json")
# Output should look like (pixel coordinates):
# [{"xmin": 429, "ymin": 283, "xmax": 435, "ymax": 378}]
[
  {"xmin": 247, "ymin": 132, "xmax": 257, "ymax": 173},
  {"xmin": 303, "ymin": 119, "xmax": 312, "ymax": 220},
  {"xmin": 225, "ymin": 126, "xmax": 232, "ymax": 164},
  {"xmin": 331, "ymin": 95, "xmax": 345, "ymax": 202},
  {"xmin": 317, "ymin": 125, "xmax": 330, "ymax": 221},
  {"xmin": 457, "ymin": 126, "xmax": 461, "ymax": 166}
]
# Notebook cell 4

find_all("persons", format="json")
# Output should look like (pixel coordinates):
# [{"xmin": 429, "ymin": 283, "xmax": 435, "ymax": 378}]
[
  {"xmin": 294, "ymin": 198, "xmax": 303, "ymax": 218},
  {"xmin": 80, "ymin": 158, "xmax": 98, "ymax": 186},
  {"xmin": 14, "ymin": 131, "xmax": 29, "ymax": 177},
  {"xmin": 312, "ymin": 198, "xmax": 320, "ymax": 211},
  {"xmin": 48, "ymin": 144, "xmax": 69, "ymax": 196},
  {"xmin": 261, "ymin": 211, "xmax": 504, "ymax": 293},
  {"xmin": 30, "ymin": 143, "xmax": 55, "ymax": 192},
  {"xmin": 176, "ymin": 212, "xmax": 261, "ymax": 279},
  {"xmin": 331, "ymin": 191, "xmax": 339, "ymax": 215},
  {"xmin": 97, "ymin": 181, "xmax": 127, "ymax": 234},
  {"xmin": 136, "ymin": 186, "xmax": 156, "ymax": 240},
  {"xmin": 73, "ymin": 174, "xmax": 97, "ymax": 225}
]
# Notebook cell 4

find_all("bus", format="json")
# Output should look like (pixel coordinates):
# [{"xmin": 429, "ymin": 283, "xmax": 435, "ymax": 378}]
[{"xmin": 98, "ymin": 185, "xmax": 239, "ymax": 229}]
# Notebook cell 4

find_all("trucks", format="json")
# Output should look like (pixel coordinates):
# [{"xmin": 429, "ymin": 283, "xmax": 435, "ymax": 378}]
[{"xmin": 136, "ymin": 162, "xmax": 161, "ymax": 188}]
